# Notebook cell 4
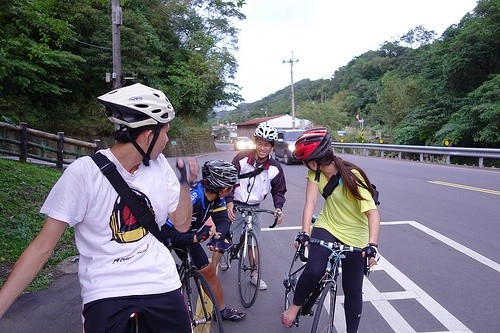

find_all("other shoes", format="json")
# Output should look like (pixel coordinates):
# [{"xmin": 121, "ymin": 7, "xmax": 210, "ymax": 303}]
[{"xmin": 282, "ymin": 308, "xmax": 295, "ymax": 328}]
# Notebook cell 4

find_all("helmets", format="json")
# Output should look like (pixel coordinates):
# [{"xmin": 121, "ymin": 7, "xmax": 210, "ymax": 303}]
[
  {"xmin": 201, "ymin": 159, "xmax": 240, "ymax": 193},
  {"xmin": 253, "ymin": 124, "xmax": 279, "ymax": 143},
  {"xmin": 292, "ymin": 127, "xmax": 333, "ymax": 163},
  {"xmin": 97, "ymin": 83, "xmax": 176, "ymax": 132}
]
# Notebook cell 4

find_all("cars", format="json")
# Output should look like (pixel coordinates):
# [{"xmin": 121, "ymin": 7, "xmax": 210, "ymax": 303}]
[{"xmin": 234, "ymin": 137, "xmax": 254, "ymax": 151}]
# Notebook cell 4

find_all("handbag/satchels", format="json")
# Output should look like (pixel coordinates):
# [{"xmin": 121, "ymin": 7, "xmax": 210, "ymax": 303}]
[{"xmin": 371, "ymin": 183, "xmax": 381, "ymax": 206}]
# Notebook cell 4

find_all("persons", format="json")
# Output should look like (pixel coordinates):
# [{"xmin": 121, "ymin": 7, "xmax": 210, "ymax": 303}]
[
  {"xmin": 212, "ymin": 126, "xmax": 287, "ymax": 290},
  {"xmin": 161, "ymin": 160, "xmax": 246, "ymax": 322},
  {"xmin": 0, "ymin": 83, "xmax": 201, "ymax": 333},
  {"xmin": 281, "ymin": 127, "xmax": 381, "ymax": 333}
]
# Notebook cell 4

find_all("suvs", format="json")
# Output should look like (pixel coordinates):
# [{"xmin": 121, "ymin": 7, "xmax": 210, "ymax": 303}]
[{"xmin": 273, "ymin": 129, "xmax": 307, "ymax": 165}]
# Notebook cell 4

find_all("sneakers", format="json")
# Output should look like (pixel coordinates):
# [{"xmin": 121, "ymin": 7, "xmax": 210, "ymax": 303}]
[
  {"xmin": 249, "ymin": 273, "xmax": 267, "ymax": 289},
  {"xmin": 220, "ymin": 256, "xmax": 228, "ymax": 271},
  {"xmin": 212, "ymin": 306, "xmax": 245, "ymax": 322}
]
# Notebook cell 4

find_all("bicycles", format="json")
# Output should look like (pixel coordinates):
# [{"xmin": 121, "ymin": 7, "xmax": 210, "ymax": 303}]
[
  {"xmin": 283, "ymin": 214, "xmax": 377, "ymax": 333},
  {"xmin": 162, "ymin": 235, "xmax": 224, "ymax": 333},
  {"xmin": 219, "ymin": 204, "xmax": 282, "ymax": 309}
]
